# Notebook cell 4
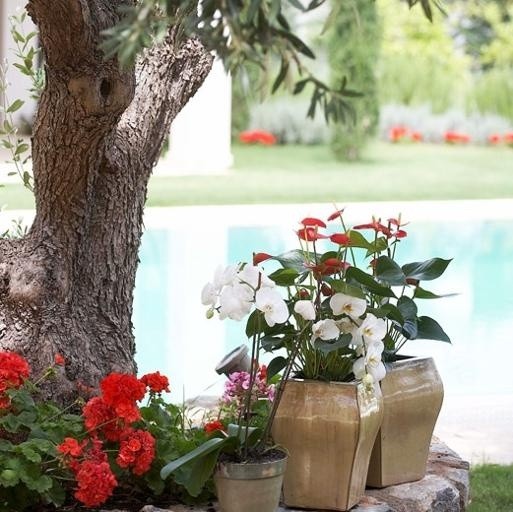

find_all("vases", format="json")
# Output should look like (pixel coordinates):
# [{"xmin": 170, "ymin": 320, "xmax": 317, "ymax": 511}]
[{"xmin": 213, "ymin": 351, "xmax": 443, "ymax": 512}]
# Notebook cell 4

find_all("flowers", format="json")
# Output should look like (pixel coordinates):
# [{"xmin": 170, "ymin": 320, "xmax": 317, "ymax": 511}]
[{"xmin": 157, "ymin": 200, "xmax": 454, "ymax": 498}]
